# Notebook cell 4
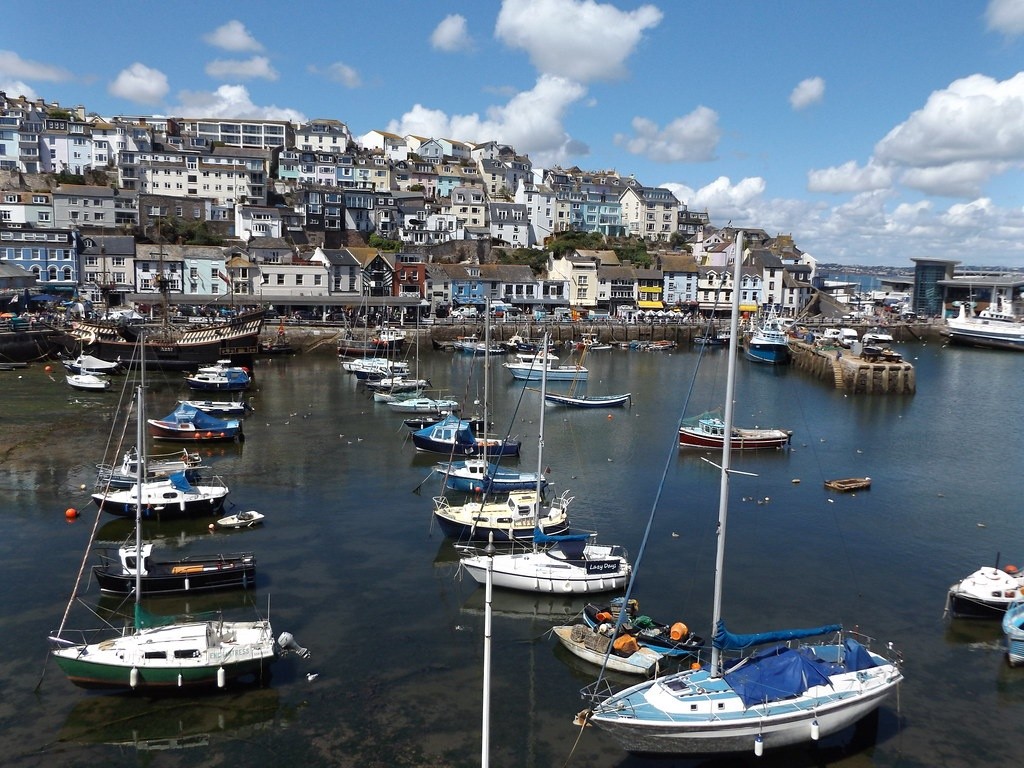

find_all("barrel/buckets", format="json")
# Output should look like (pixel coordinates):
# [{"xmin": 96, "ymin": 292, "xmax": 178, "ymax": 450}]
[{"xmin": 670, "ymin": 624, "xmax": 684, "ymax": 640}]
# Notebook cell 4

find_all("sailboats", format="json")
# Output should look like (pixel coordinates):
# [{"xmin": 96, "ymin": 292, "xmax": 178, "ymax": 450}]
[
  {"xmin": 45, "ymin": 317, "xmax": 310, "ymax": 690},
  {"xmin": 571, "ymin": 231, "xmax": 919, "ymax": 759},
  {"xmin": 453, "ymin": 330, "xmax": 635, "ymax": 597}
]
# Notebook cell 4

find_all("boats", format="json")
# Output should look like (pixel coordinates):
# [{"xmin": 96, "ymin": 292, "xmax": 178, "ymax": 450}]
[
  {"xmin": 63, "ymin": 220, "xmax": 743, "ymax": 544},
  {"xmin": 676, "ymin": 419, "xmax": 793, "ymax": 452},
  {"xmin": 742, "ymin": 299, "xmax": 791, "ymax": 366},
  {"xmin": 822, "ymin": 476, "xmax": 872, "ymax": 493},
  {"xmin": 217, "ymin": 510, "xmax": 264, "ymax": 528},
  {"xmin": 550, "ymin": 601, "xmax": 705, "ymax": 676},
  {"xmin": 90, "ymin": 537, "xmax": 258, "ymax": 599},
  {"xmin": 937, "ymin": 284, "xmax": 1024, "ymax": 352},
  {"xmin": 1002, "ymin": 600, "xmax": 1024, "ymax": 667},
  {"xmin": 942, "ymin": 556, "xmax": 1024, "ymax": 622}
]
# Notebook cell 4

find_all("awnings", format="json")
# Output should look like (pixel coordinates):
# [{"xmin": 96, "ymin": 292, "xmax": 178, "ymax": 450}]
[
  {"xmin": 638, "ymin": 301, "xmax": 664, "ymax": 308},
  {"xmin": 740, "ymin": 306, "xmax": 757, "ymax": 311}
]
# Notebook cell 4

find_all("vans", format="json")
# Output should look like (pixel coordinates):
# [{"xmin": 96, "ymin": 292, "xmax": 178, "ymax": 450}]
[
  {"xmin": 450, "ymin": 307, "xmax": 477, "ymax": 318},
  {"xmin": 838, "ymin": 328, "xmax": 858, "ymax": 349}
]
